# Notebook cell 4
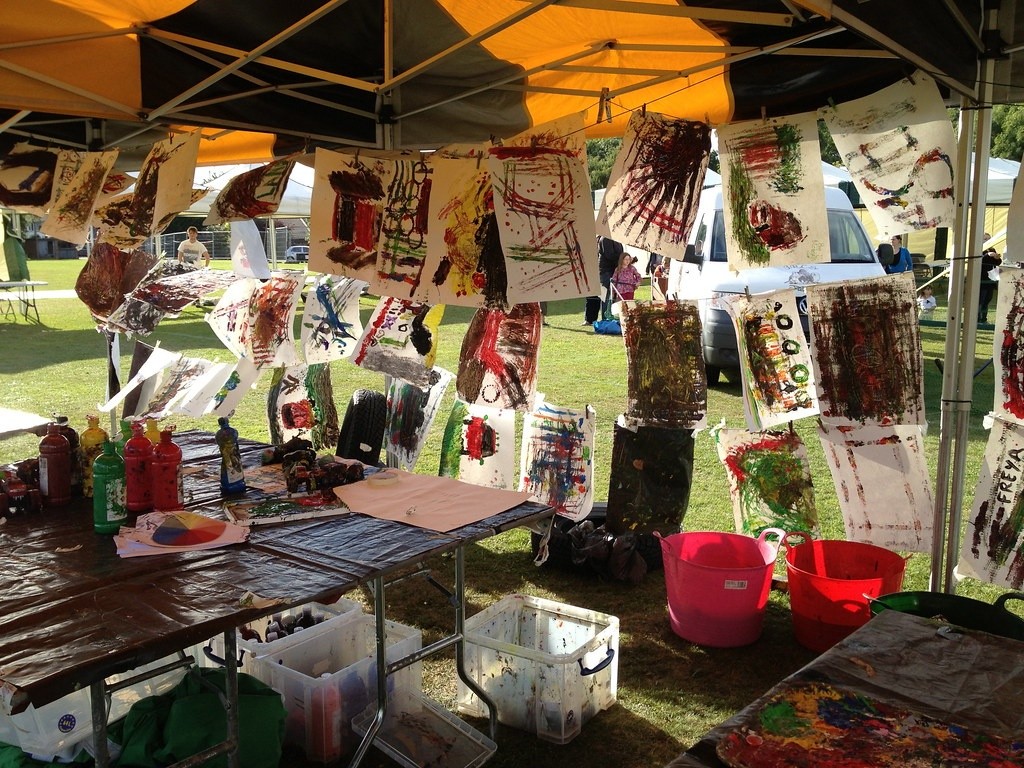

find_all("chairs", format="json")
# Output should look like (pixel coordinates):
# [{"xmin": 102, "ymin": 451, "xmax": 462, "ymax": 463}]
[{"xmin": 911, "ymin": 252, "xmax": 935, "ymax": 289}]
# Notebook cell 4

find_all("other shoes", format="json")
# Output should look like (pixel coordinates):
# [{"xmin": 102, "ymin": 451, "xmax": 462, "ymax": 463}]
[{"xmin": 581, "ymin": 321, "xmax": 594, "ymax": 325}]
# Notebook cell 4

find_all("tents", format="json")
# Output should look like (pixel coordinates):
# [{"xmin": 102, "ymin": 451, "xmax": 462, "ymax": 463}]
[
  {"xmin": 595, "ymin": 168, "xmax": 722, "ymax": 211},
  {"xmin": 0, "ymin": 0, "xmax": 1024, "ymax": 173},
  {"xmin": 839, "ymin": 152, "xmax": 1022, "ymax": 209},
  {"xmin": 820, "ymin": 159, "xmax": 853, "ymax": 185},
  {"xmin": 109, "ymin": 160, "xmax": 315, "ymax": 215}
]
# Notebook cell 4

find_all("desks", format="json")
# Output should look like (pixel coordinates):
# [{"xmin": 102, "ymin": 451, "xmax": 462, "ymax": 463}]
[
  {"xmin": 0, "ymin": 281, "xmax": 50, "ymax": 323},
  {"xmin": 0, "ymin": 429, "xmax": 558, "ymax": 768},
  {"xmin": 666, "ymin": 611, "xmax": 1024, "ymax": 768}
]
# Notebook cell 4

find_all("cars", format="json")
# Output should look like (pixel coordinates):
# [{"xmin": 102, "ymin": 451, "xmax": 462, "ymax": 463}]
[{"xmin": 285, "ymin": 246, "xmax": 310, "ymax": 261}]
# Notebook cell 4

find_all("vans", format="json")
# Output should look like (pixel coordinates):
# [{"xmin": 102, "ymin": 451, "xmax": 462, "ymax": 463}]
[{"xmin": 665, "ymin": 187, "xmax": 888, "ymax": 387}]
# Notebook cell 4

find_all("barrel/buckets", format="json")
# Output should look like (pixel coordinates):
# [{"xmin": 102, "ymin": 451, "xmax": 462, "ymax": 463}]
[
  {"xmin": 652, "ymin": 527, "xmax": 785, "ymax": 648},
  {"xmin": 781, "ymin": 531, "xmax": 913, "ymax": 654}
]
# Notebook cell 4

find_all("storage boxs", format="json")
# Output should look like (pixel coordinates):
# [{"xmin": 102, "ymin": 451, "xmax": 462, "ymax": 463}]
[
  {"xmin": 458, "ymin": 593, "xmax": 620, "ymax": 744},
  {"xmin": 0, "ymin": 592, "xmax": 497, "ymax": 768},
  {"xmin": 532, "ymin": 502, "xmax": 607, "ymax": 570}
]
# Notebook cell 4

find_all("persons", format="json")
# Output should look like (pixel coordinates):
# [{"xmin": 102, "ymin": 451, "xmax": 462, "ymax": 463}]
[
  {"xmin": 177, "ymin": 227, "xmax": 210, "ymax": 308},
  {"xmin": 882, "ymin": 235, "xmax": 913, "ymax": 275},
  {"xmin": 580, "ymin": 234, "xmax": 624, "ymax": 326},
  {"xmin": 916, "ymin": 285, "xmax": 937, "ymax": 320},
  {"xmin": 654, "ymin": 256, "xmax": 671, "ymax": 279},
  {"xmin": 612, "ymin": 252, "xmax": 643, "ymax": 301},
  {"xmin": 977, "ymin": 232, "xmax": 1002, "ymax": 325},
  {"xmin": 645, "ymin": 252, "xmax": 664, "ymax": 275}
]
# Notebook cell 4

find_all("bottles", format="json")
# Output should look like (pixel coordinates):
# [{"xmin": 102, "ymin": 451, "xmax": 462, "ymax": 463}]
[
  {"xmin": 215, "ymin": 417, "xmax": 245, "ymax": 494},
  {"xmin": 38, "ymin": 413, "xmax": 184, "ymax": 534}
]
